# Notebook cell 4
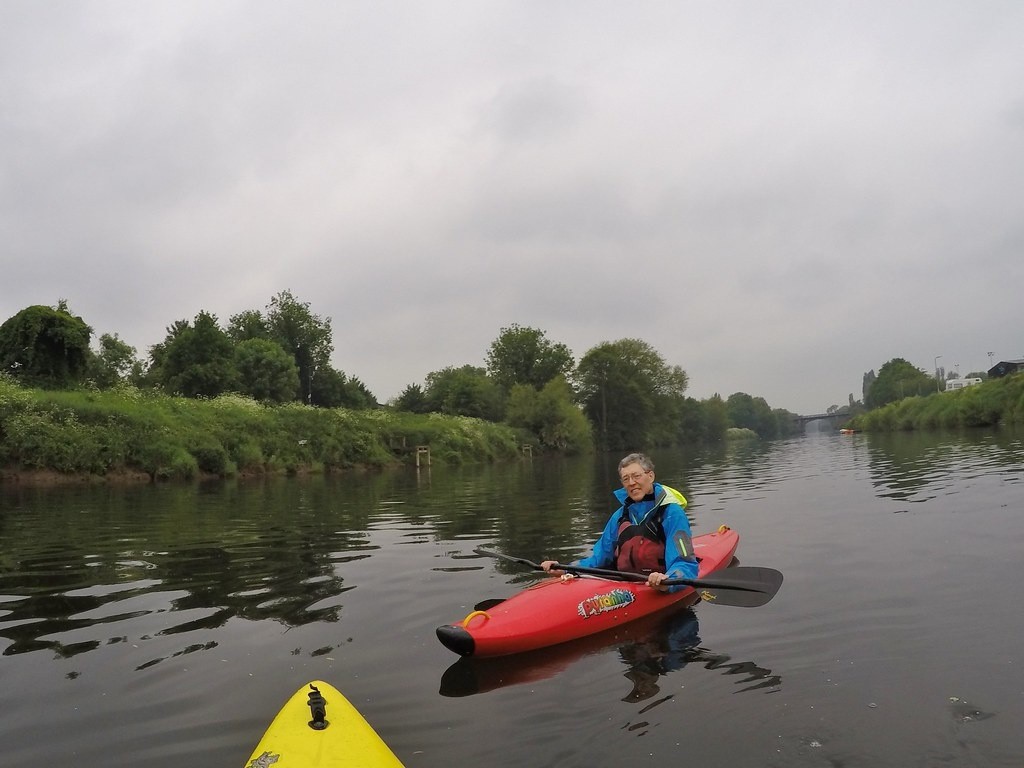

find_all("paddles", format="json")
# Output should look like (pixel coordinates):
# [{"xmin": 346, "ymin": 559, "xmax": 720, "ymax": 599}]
[{"xmin": 473, "ymin": 547, "xmax": 783, "ymax": 607}]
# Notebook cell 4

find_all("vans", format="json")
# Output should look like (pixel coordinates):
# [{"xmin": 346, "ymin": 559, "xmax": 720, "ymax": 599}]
[{"xmin": 945, "ymin": 378, "xmax": 982, "ymax": 392}]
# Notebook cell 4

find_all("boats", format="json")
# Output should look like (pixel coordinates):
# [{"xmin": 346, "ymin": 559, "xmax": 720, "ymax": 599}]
[
  {"xmin": 434, "ymin": 524, "xmax": 740, "ymax": 660},
  {"xmin": 839, "ymin": 428, "xmax": 862, "ymax": 435}
]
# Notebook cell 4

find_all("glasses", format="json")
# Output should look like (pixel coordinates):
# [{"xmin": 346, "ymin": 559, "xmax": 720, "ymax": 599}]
[{"xmin": 620, "ymin": 470, "xmax": 651, "ymax": 483}]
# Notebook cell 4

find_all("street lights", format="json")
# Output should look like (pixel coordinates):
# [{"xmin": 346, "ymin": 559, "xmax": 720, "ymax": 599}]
[
  {"xmin": 934, "ymin": 354, "xmax": 941, "ymax": 391},
  {"xmin": 987, "ymin": 350, "xmax": 995, "ymax": 368},
  {"xmin": 955, "ymin": 363, "xmax": 959, "ymax": 378}
]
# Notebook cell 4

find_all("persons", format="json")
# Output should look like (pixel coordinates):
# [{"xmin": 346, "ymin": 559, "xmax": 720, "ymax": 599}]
[{"xmin": 541, "ymin": 453, "xmax": 700, "ymax": 593}]
